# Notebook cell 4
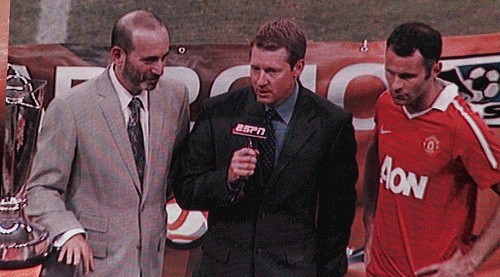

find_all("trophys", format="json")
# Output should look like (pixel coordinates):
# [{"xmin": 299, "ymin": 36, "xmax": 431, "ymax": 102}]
[{"xmin": 0, "ymin": 61, "xmax": 50, "ymax": 269}]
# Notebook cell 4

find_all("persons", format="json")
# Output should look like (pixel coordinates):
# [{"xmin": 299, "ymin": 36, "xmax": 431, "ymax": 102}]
[
  {"xmin": 173, "ymin": 20, "xmax": 360, "ymax": 277},
  {"xmin": 24, "ymin": 11, "xmax": 190, "ymax": 277},
  {"xmin": 363, "ymin": 21, "xmax": 500, "ymax": 277}
]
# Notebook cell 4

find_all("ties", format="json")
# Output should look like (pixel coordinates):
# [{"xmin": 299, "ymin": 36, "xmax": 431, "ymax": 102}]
[
  {"xmin": 127, "ymin": 97, "xmax": 145, "ymax": 186},
  {"xmin": 258, "ymin": 108, "xmax": 280, "ymax": 188}
]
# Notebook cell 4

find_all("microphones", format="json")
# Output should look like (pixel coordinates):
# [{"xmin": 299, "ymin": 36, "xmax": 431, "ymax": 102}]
[{"xmin": 235, "ymin": 108, "xmax": 264, "ymax": 197}]
